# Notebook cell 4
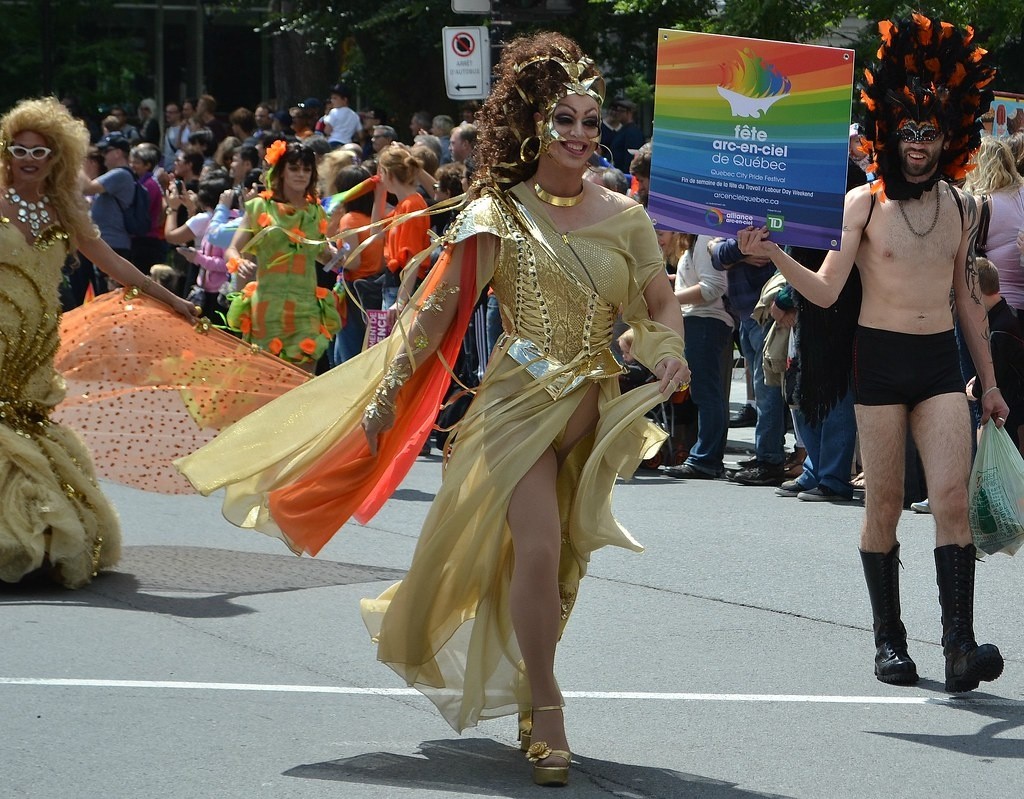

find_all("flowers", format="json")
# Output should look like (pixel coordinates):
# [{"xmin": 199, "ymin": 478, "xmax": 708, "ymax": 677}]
[
  {"xmin": 243, "ymin": 280, "xmax": 257, "ymax": 297},
  {"xmin": 225, "ymin": 257, "xmax": 239, "ymax": 273},
  {"xmin": 268, "ymin": 336, "xmax": 283, "ymax": 355},
  {"xmin": 257, "ymin": 190, "xmax": 274, "ymax": 202},
  {"xmin": 318, "ymin": 219, "xmax": 328, "ymax": 236},
  {"xmin": 264, "ymin": 140, "xmax": 287, "ymax": 166},
  {"xmin": 300, "ymin": 337, "xmax": 316, "ymax": 354},
  {"xmin": 257, "ymin": 213, "xmax": 271, "ymax": 228},
  {"xmin": 316, "ymin": 286, "xmax": 328, "ymax": 298},
  {"xmin": 240, "ymin": 317, "xmax": 252, "ymax": 335},
  {"xmin": 307, "ymin": 194, "xmax": 321, "ymax": 205},
  {"xmin": 289, "ymin": 228, "xmax": 306, "ymax": 244},
  {"xmin": 320, "ymin": 324, "xmax": 331, "ymax": 339}
]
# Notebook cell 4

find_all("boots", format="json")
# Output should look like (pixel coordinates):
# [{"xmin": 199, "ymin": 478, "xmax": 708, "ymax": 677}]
[
  {"xmin": 863, "ymin": 545, "xmax": 920, "ymax": 688},
  {"xmin": 780, "ymin": 442, "xmax": 808, "ymax": 471},
  {"xmin": 928, "ymin": 535, "xmax": 1000, "ymax": 697}
]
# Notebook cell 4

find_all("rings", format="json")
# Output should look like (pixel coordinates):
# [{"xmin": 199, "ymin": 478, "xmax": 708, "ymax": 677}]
[{"xmin": 680, "ymin": 380, "xmax": 690, "ymax": 391}]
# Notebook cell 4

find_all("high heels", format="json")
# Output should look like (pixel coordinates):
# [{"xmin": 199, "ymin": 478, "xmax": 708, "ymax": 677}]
[
  {"xmin": 523, "ymin": 707, "xmax": 574, "ymax": 787},
  {"xmin": 508, "ymin": 683, "xmax": 546, "ymax": 753}
]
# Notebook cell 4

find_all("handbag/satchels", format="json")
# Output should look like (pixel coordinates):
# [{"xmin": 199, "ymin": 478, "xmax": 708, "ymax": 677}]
[
  {"xmin": 962, "ymin": 402, "xmax": 1024, "ymax": 559},
  {"xmin": 183, "ymin": 282, "xmax": 208, "ymax": 314}
]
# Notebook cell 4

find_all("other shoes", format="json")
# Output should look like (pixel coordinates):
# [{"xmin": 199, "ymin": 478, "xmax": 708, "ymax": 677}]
[
  {"xmin": 738, "ymin": 456, "xmax": 758, "ymax": 467},
  {"xmin": 657, "ymin": 459, "xmax": 715, "ymax": 481},
  {"xmin": 792, "ymin": 484, "xmax": 855, "ymax": 505},
  {"xmin": 772, "ymin": 471, "xmax": 816, "ymax": 498},
  {"xmin": 912, "ymin": 493, "xmax": 931, "ymax": 513}
]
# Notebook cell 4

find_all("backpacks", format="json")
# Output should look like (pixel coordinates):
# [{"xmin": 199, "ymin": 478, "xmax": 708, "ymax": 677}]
[{"xmin": 106, "ymin": 167, "xmax": 152, "ymax": 241}]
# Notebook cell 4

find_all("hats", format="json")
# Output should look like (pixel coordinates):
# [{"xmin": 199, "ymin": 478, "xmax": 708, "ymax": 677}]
[
  {"xmin": 267, "ymin": 110, "xmax": 293, "ymax": 126},
  {"xmin": 331, "ymin": 82, "xmax": 354, "ymax": 99},
  {"xmin": 297, "ymin": 97, "xmax": 321, "ymax": 111},
  {"xmin": 93, "ymin": 134, "xmax": 130, "ymax": 153},
  {"xmin": 848, "ymin": 123, "xmax": 862, "ymax": 139}
]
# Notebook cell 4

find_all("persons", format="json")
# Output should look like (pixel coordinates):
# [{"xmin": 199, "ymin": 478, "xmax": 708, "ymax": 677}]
[
  {"xmin": 0, "ymin": 93, "xmax": 203, "ymax": 590},
  {"xmin": 360, "ymin": 34, "xmax": 693, "ymax": 790},
  {"xmin": 592, "ymin": 91, "xmax": 1021, "ymax": 530},
  {"xmin": 54, "ymin": 80, "xmax": 507, "ymax": 462},
  {"xmin": 737, "ymin": 71, "xmax": 1010, "ymax": 693}
]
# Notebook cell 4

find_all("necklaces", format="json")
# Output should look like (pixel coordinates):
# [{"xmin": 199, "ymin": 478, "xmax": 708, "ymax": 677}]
[
  {"xmin": 532, "ymin": 174, "xmax": 586, "ymax": 207},
  {"xmin": 4, "ymin": 184, "xmax": 52, "ymax": 238}
]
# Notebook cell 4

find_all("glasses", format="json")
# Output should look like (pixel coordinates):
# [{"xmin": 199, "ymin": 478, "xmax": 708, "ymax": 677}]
[
  {"xmin": 5, "ymin": 144, "xmax": 54, "ymax": 160},
  {"xmin": 175, "ymin": 157, "xmax": 191, "ymax": 166},
  {"xmin": 432, "ymin": 182, "xmax": 443, "ymax": 192},
  {"xmin": 165, "ymin": 110, "xmax": 182, "ymax": 116},
  {"xmin": 371, "ymin": 133, "xmax": 384, "ymax": 140},
  {"xmin": 459, "ymin": 174, "xmax": 469, "ymax": 180},
  {"xmin": 140, "ymin": 106, "xmax": 149, "ymax": 112}
]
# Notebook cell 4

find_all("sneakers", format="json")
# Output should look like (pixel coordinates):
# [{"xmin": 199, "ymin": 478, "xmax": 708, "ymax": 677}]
[
  {"xmin": 723, "ymin": 460, "xmax": 786, "ymax": 487},
  {"xmin": 728, "ymin": 403, "xmax": 755, "ymax": 430}
]
